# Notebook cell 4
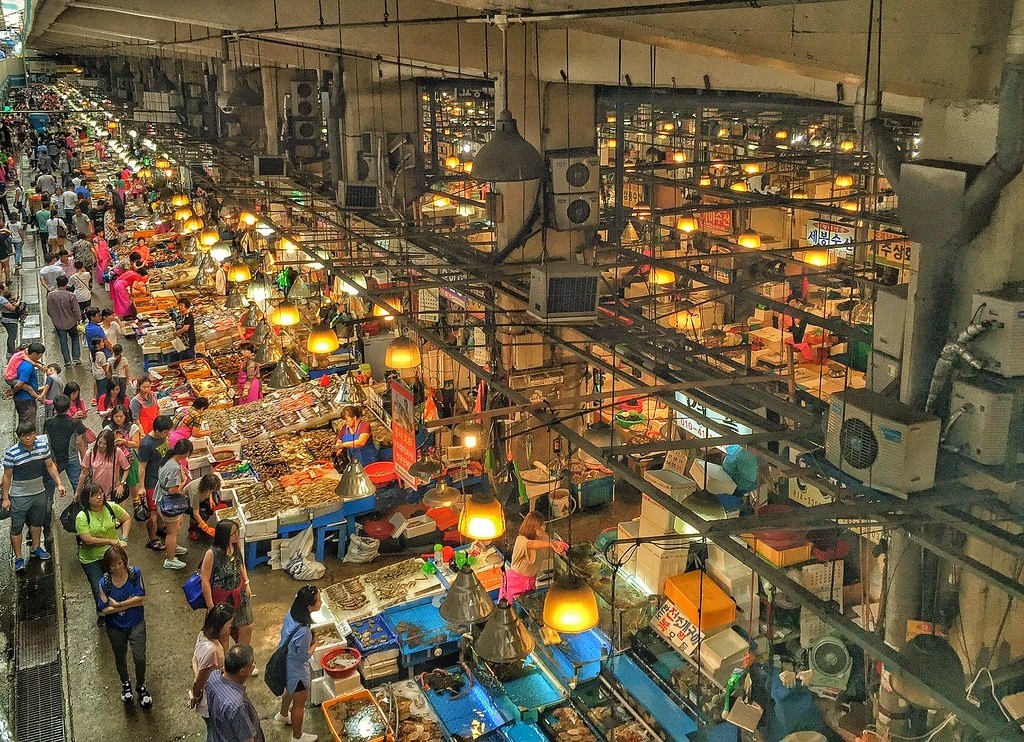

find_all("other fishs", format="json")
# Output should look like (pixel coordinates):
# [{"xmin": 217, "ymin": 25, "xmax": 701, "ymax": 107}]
[{"xmin": 327, "ymin": 560, "xmax": 427, "ymax": 610}]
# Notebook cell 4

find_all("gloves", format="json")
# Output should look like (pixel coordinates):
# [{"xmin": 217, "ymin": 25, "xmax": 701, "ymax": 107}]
[{"xmin": 199, "ymin": 521, "xmax": 216, "ymax": 537}]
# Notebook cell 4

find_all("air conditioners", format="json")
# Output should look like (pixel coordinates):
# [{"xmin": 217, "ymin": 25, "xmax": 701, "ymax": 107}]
[
  {"xmin": 524, "ymin": 153, "xmax": 601, "ymax": 319},
  {"xmin": 106, "ymin": 77, "xmax": 134, "ymax": 99},
  {"xmin": 254, "ymin": 152, "xmax": 288, "ymax": 180},
  {"xmin": 948, "ymin": 289, "xmax": 1024, "ymax": 466},
  {"xmin": 336, "ymin": 132, "xmax": 414, "ymax": 207},
  {"xmin": 825, "ymin": 283, "xmax": 942, "ymax": 493},
  {"xmin": 290, "ymin": 82, "xmax": 321, "ymax": 139}
]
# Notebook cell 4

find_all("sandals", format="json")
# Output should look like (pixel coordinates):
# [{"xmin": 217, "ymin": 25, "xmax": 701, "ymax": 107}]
[{"xmin": 146, "ymin": 539, "xmax": 166, "ymax": 550}]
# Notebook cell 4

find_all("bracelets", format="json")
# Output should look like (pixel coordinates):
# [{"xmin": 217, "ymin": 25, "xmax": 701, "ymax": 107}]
[{"xmin": 245, "ymin": 579, "xmax": 250, "ymax": 584}]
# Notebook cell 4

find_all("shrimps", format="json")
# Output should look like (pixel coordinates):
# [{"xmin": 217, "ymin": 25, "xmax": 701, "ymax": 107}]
[
  {"xmin": 265, "ymin": 394, "xmax": 329, "ymax": 432},
  {"xmin": 237, "ymin": 468, "xmax": 342, "ymax": 520}
]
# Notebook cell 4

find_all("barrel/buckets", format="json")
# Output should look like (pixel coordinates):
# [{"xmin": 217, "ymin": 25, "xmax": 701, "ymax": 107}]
[{"xmin": 548, "ymin": 488, "xmax": 570, "ymax": 518}]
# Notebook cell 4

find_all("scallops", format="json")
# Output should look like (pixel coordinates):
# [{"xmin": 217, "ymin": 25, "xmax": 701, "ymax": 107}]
[{"xmin": 378, "ymin": 692, "xmax": 442, "ymax": 742}]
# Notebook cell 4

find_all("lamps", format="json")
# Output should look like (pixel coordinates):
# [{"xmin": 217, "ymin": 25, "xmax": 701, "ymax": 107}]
[
  {"xmin": 268, "ymin": 266, "xmax": 421, "ymax": 497},
  {"xmin": 426, "ymin": 414, "xmax": 599, "ymax": 661},
  {"xmin": 422, "ymin": 95, "xmax": 485, "ymax": 217},
  {"xmin": 53, "ymin": 86, "xmax": 271, "ymax": 360},
  {"xmin": 608, "ymin": 111, "xmax": 861, "ymax": 330},
  {"xmin": 892, "ymin": 555, "xmax": 965, "ymax": 709},
  {"xmin": 674, "ymin": 404, "xmax": 727, "ymax": 544}
]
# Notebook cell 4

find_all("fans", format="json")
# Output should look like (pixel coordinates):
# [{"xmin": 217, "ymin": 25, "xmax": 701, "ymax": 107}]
[{"xmin": 809, "ymin": 637, "xmax": 853, "ymax": 690}]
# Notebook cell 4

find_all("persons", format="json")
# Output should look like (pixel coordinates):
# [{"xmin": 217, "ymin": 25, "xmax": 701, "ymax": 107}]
[
  {"xmin": 237, "ymin": 307, "xmax": 257, "ymax": 340},
  {"xmin": 239, "ymin": 343, "xmax": 263, "ymax": 404},
  {"xmin": 201, "ymin": 645, "xmax": 265, "ymax": 742},
  {"xmin": 498, "ymin": 512, "xmax": 569, "ymax": 605},
  {"xmin": 335, "ymin": 407, "xmax": 378, "ymax": 467},
  {"xmin": 482, "ymin": 184, "xmax": 488, "ymax": 199},
  {"xmin": 275, "ymin": 586, "xmax": 322, "ymax": 742},
  {"xmin": 778, "ymin": 295, "xmax": 798, "ymax": 331},
  {"xmin": 201, "ymin": 519, "xmax": 260, "ymax": 676},
  {"xmin": 215, "ymin": 261, "xmax": 236, "ymax": 296},
  {"xmin": 98, "ymin": 546, "xmax": 153, "ymax": 707},
  {"xmin": 0, "ymin": 91, "xmax": 220, "ymax": 627},
  {"xmin": 192, "ymin": 603, "xmax": 233, "ymax": 725}
]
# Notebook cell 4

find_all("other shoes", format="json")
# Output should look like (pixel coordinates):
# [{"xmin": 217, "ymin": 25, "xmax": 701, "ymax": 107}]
[
  {"xmin": 73, "ymin": 357, "xmax": 82, "ymax": 364},
  {"xmin": 6, "ymin": 279, "xmax": 13, "ymax": 285},
  {"xmin": 66, "ymin": 362, "xmax": 72, "ymax": 369}
]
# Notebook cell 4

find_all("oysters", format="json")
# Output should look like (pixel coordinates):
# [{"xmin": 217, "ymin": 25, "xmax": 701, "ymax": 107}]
[{"xmin": 352, "ymin": 620, "xmax": 388, "ymax": 648}]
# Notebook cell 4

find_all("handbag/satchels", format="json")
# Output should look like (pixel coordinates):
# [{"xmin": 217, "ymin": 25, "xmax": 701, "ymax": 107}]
[
  {"xmin": 13, "ymin": 200, "xmax": 23, "ymax": 208},
  {"xmin": 161, "ymin": 493, "xmax": 188, "ymax": 517},
  {"xmin": 182, "ymin": 551, "xmax": 217, "ymax": 610},
  {"xmin": 60, "ymin": 501, "xmax": 84, "ymax": 533},
  {"xmin": 213, "ymin": 587, "xmax": 241, "ymax": 609},
  {"xmin": 263, "ymin": 646, "xmax": 289, "ymax": 697},
  {"xmin": 83, "ymin": 428, "xmax": 96, "ymax": 444},
  {"xmin": 110, "ymin": 484, "xmax": 129, "ymax": 504},
  {"xmin": 134, "ymin": 496, "xmax": 151, "ymax": 521},
  {"xmin": 57, "ymin": 219, "xmax": 66, "ymax": 238}
]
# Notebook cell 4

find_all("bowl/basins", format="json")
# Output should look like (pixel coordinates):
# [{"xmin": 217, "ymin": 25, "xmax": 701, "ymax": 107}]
[
  {"xmin": 208, "ymin": 450, "xmax": 242, "ymax": 472},
  {"xmin": 615, "ymin": 411, "xmax": 644, "ymax": 429},
  {"xmin": 362, "ymin": 520, "xmax": 397, "ymax": 541},
  {"xmin": 618, "ymin": 395, "xmax": 648, "ymax": 412},
  {"xmin": 363, "ymin": 461, "xmax": 396, "ymax": 484},
  {"xmin": 319, "ymin": 647, "xmax": 362, "ymax": 679}
]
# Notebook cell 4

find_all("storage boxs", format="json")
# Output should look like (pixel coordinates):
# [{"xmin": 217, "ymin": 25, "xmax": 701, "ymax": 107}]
[
  {"xmin": 617, "ymin": 460, "xmax": 849, "ymax": 674},
  {"xmin": 310, "ymin": 621, "xmax": 396, "ymax": 741},
  {"xmin": 206, "ymin": 437, "xmax": 277, "ymax": 539}
]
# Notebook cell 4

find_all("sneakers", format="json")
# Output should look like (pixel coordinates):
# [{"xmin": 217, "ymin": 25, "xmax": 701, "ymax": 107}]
[
  {"xmin": 136, "ymin": 685, "xmax": 152, "ymax": 706},
  {"xmin": 121, "ymin": 679, "xmax": 134, "ymax": 702},
  {"xmin": 274, "ymin": 711, "xmax": 292, "ymax": 724},
  {"xmin": 291, "ymin": 733, "xmax": 317, "ymax": 742},
  {"xmin": 15, "ymin": 558, "xmax": 25, "ymax": 572},
  {"xmin": 163, "ymin": 557, "xmax": 186, "ymax": 570},
  {"xmin": 30, "ymin": 546, "xmax": 51, "ymax": 559},
  {"xmin": 166, "ymin": 545, "xmax": 188, "ymax": 555},
  {"xmin": 41, "ymin": 399, "xmax": 54, "ymax": 405}
]
